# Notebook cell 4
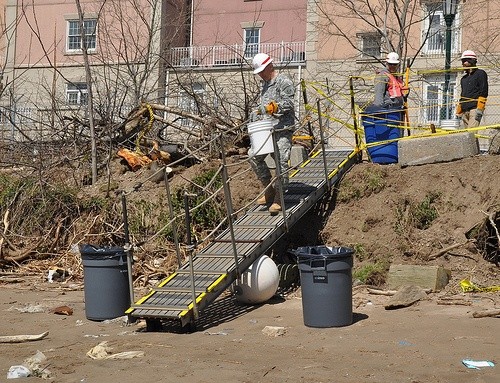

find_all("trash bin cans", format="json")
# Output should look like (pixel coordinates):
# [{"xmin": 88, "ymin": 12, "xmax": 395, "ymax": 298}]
[
  {"xmin": 361, "ymin": 108, "xmax": 400, "ymax": 164},
  {"xmin": 81, "ymin": 245, "xmax": 130, "ymax": 321},
  {"xmin": 295, "ymin": 245, "xmax": 354, "ymax": 328}
]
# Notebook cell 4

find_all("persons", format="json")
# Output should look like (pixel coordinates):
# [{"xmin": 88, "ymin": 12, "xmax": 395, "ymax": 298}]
[
  {"xmin": 249, "ymin": 53, "xmax": 298, "ymax": 215},
  {"xmin": 457, "ymin": 50, "xmax": 489, "ymax": 135},
  {"xmin": 372, "ymin": 52, "xmax": 408, "ymax": 138}
]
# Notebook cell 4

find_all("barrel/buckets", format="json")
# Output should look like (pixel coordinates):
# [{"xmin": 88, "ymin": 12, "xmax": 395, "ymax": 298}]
[
  {"xmin": 441, "ymin": 119, "xmax": 460, "ymax": 130},
  {"xmin": 246, "ymin": 120, "xmax": 275, "ymax": 156}
]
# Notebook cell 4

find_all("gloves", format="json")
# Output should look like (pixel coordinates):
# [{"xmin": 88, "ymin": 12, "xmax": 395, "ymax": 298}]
[
  {"xmin": 475, "ymin": 110, "xmax": 483, "ymax": 120},
  {"xmin": 256, "ymin": 105, "xmax": 273, "ymax": 116}
]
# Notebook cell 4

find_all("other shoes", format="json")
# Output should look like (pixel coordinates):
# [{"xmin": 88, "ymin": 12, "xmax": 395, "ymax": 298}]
[
  {"xmin": 258, "ymin": 191, "xmax": 274, "ymax": 205},
  {"xmin": 269, "ymin": 202, "xmax": 281, "ymax": 213}
]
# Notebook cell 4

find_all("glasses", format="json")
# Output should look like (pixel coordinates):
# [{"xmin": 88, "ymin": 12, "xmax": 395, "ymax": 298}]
[{"xmin": 387, "ymin": 62, "xmax": 397, "ymax": 66}]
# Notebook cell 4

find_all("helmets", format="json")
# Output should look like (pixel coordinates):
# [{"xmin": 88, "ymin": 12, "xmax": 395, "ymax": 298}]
[
  {"xmin": 460, "ymin": 50, "xmax": 477, "ymax": 60},
  {"xmin": 252, "ymin": 53, "xmax": 273, "ymax": 75},
  {"xmin": 386, "ymin": 52, "xmax": 400, "ymax": 64}
]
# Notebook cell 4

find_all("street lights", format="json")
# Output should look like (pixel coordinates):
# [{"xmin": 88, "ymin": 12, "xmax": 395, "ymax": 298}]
[{"xmin": 440, "ymin": 0, "xmax": 461, "ymax": 119}]
